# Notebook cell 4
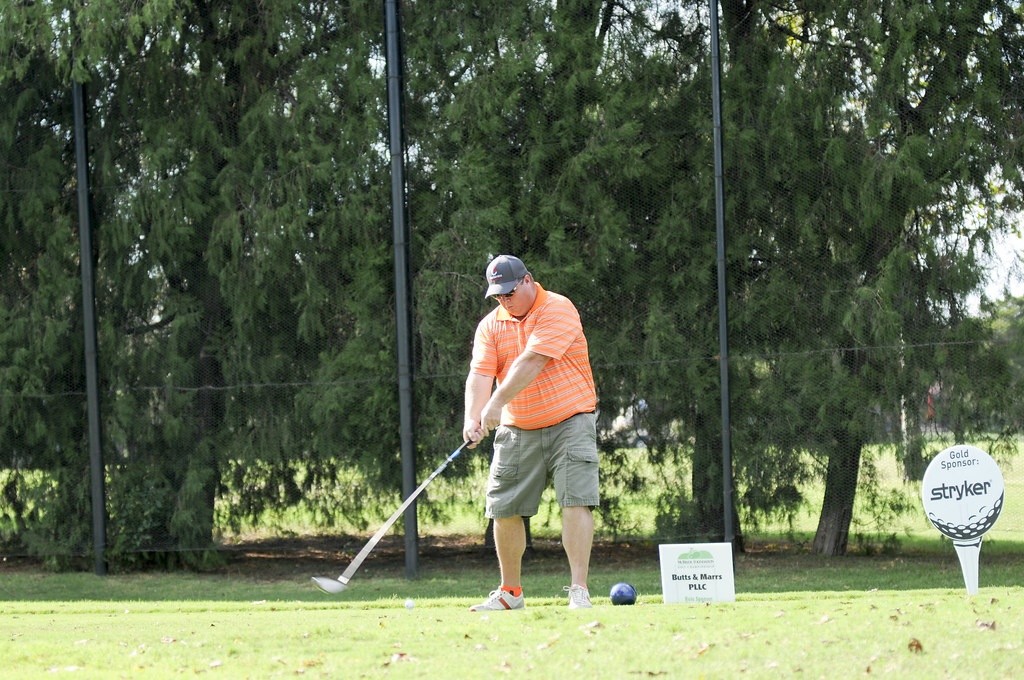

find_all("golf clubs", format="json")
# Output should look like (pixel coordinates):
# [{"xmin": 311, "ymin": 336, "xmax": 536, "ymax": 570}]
[{"xmin": 309, "ymin": 440, "xmax": 472, "ymax": 593}]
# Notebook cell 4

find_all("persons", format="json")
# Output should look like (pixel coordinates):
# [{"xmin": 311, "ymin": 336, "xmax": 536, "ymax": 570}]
[{"xmin": 463, "ymin": 255, "xmax": 602, "ymax": 612}]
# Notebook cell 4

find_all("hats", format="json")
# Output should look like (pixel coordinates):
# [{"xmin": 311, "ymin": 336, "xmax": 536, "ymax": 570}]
[{"xmin": 484, "ymin": 255, "xmax": 528, "ymax": 299}]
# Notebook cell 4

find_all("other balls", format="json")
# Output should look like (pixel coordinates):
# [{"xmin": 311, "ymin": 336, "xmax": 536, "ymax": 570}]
[{"xmin": 609, "ymin": 582, "xmax": 637, "ymax": 604}]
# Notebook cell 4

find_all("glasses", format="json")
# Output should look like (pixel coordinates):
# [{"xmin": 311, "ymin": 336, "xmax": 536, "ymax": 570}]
[{"xmin": 491, "ymin": 280, "xmax": 523, "ymax": 299}]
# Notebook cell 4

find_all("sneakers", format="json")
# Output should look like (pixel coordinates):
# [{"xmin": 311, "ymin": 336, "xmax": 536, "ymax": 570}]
[
  {"xmin": 563, "ymin": 584, "xmax": 593, "ymax": 609},
  {"xmin": 469, "ymin": 586, "xmax": 524, "ymax": 612}
]
